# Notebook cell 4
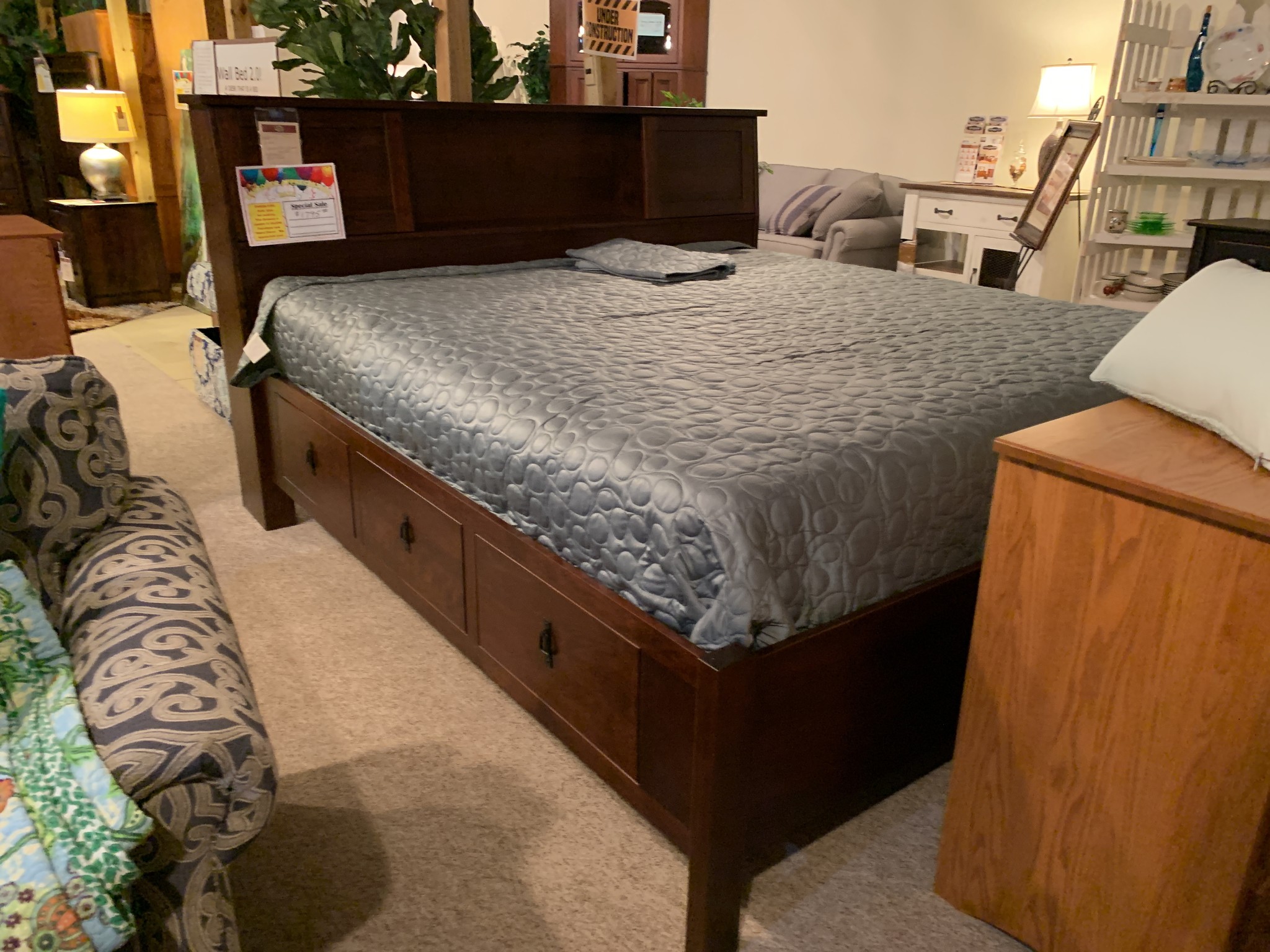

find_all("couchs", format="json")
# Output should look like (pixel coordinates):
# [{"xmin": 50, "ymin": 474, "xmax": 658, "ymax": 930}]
[
  {"xmin": 0, "ymin": 352, "xmax": 275, "ymax": 952},
  {"xmin": 758, "ymin": 164, "xmax": 909, "ymax": 272}
]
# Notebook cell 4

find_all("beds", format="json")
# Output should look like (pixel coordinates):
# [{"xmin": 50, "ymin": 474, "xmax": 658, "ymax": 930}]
[{"xmin": 178, "ymin": 95, "xmax": 1146, "ymax": 951}]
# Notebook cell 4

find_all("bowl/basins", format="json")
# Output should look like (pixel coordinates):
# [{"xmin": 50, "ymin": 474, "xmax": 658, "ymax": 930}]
[
  {"xmin": 1121, "ymin": 288, "xmax": 1163, "ymax": 302},
  {"xmin": 1122, "ymin": 282, "xmax": 1163, "ymax": 293},
  {"xmin": 1136, "ymin": 81, "xmax": 1162, "ymax": 91},
  {"xmin": 1124, "ymin": 275, "xmax": 1163, "ymax": 290}
]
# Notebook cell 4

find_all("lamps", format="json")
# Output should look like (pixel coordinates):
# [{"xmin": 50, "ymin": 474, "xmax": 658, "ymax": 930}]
[
  {"xmin": 1028, "ymin": 58, "xmax": 1098, "ymax": 178},
  {"xmin": 57, "ymin": 84, "xmax": 139, "ymax": 202}
]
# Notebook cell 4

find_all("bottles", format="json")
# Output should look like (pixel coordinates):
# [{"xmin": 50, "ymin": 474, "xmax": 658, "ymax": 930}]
[
  {"xmin": 1009, "ymin": 142, "xmax": 1028, "ymax": 188},
  {"xmin": 1186, "ymin": 13, "xmax": 1211, "ymax": 92}
]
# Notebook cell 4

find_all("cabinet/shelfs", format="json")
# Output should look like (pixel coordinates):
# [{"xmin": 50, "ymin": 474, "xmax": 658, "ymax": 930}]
[
  {"xmin": 550, "ymin": 0, "xmax": 708, "ymax": 108},
  {"xmin": 49, "ymin": 198, "xmax": 172, "ymax": 308},
  {"xmin": 1072, "ymin": 88, "xmax": 1270, "ymax": 315},
  {"xmin": 895, "ymin": 182, "xmax": 1100, "ymax": 303}
]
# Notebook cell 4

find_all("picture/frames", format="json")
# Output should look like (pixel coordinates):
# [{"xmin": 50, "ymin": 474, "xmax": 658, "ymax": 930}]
[{"xmin": 1014, "ymin": 119, "xmax": 1101, "ymax": 251}]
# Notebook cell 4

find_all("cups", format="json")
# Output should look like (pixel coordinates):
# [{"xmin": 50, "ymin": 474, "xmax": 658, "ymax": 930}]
[
  {"xmin": 1165, "ymin": 78, "xmax": 1187, "ymax": 92},
  {"xmin": 1097, "ymin": 275, "xmax": 1120, "ymax": 298},
  {"xmin": 1105, "ymin": 208, "xmax": 1128, "ymax": 233},
  {"xmin": 1110, "ymin": 272, "xmax": 1127, "ymax": 294},
  {"xmin": 1129, "ymin": 270, "xmax": 1148, "ymax": 276}
]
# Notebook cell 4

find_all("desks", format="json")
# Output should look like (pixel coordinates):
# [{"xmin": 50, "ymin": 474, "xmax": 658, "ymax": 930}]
[{"xmin": 934, "ymin": 398, "xmax": 1270, "ymax": 952}]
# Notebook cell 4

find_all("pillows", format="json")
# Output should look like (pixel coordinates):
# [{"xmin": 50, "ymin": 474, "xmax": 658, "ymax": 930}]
[
  {"xmin": 813, "ymin": 172, "xmax": 884, "ymax": 241},
  {"xmin": 1088, "ymin": 259, "xmax": 1270, "ymax": 476},
  {"xmin": 765, "ymin": 183, "xmax": 843, "ymax": 236}
]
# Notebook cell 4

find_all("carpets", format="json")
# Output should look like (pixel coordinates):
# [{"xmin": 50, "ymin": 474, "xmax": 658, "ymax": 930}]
[{"xmin": 60, "ymin": 285, "xmax": 181, "ymax": 331}]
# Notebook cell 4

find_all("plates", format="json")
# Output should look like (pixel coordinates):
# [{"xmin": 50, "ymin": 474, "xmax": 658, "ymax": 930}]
[
  {"xmin": 1123, "ymin": 152, "xmax": 1270, "ymax": 164},
  {"xmin": 1200, "ymin": 23, "xmax": 1270, "ymax": 89},
  {"xmin": 1128, "ymin": 210, "xmax": 1176, "ymax": 234},
  {"xmin": 1160, "ymin": 272, "xmax": 1187, "ymax": 299}
]
